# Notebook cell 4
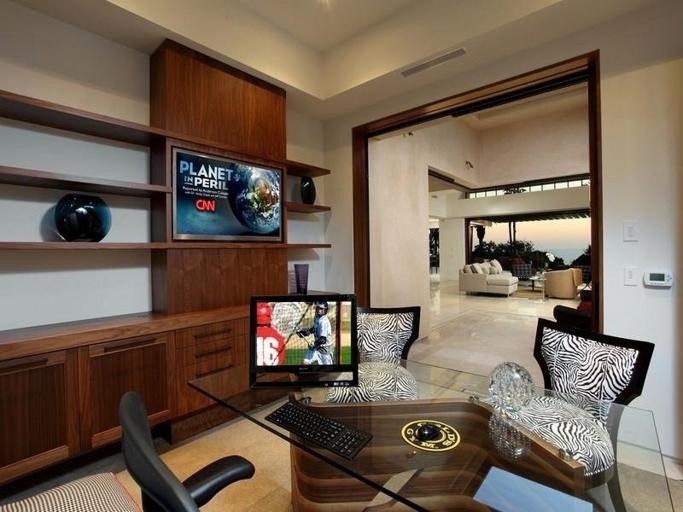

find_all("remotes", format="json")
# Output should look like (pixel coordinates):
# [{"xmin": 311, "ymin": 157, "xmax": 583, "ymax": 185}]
[{"xmin": 418, "ymin": 424, "xmax": 439, "ymax": 440}]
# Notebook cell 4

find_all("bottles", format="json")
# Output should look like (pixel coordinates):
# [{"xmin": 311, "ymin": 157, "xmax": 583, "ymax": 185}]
[{"xmin": 317, "ymin": 303, "xmax": 327, "ymax": 308}]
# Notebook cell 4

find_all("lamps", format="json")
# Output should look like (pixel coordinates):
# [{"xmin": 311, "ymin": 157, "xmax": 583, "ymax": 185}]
[
  {"xmin": 117, "ymin": 392, "xmax": 256, "ymax": 512},
  {"xmin": 487, "ymin": 316, "xmax": 654, "ymax": 510},
  {"xmin": 544, "ymin": 268, "xmax": 578, "ymax": 298},
  {"xmin": 327, "ymin": 306, "xmax": 420, "ymax": 402}
]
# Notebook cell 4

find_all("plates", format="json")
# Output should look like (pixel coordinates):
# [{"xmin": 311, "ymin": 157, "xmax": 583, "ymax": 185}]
[
  {"xmin": 0, "ymin": 88, "xmax": 165, "ymax": 249},
  {"xmin": 286, "ymin": 158, "xmax": 335, "ymax": 247},
  {"xmin": 1, "ymin": 349, "xmax": 78, "ymax": 484},
  {"xmin": 77, "ymin": 332, "xmax": 172, "ymax": 451},
  {"xmin": 169, "ymin": 320, "xmax": 247, "ymax": 418}
]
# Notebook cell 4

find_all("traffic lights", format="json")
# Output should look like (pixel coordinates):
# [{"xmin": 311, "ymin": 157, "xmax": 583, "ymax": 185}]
[{"xmin": 470, "ymin": 258, "xmax": 502, "ymax": 275}]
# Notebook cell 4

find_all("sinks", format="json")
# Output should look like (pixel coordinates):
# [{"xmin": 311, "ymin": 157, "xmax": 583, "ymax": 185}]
[{"xmin": 459, "ymin": 262, "xmax": 518, "ymax": 296}]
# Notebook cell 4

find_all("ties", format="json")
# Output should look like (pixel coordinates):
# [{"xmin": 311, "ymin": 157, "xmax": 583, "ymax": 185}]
[
  {"xmin": 247, "ymin": 293, "xmax": 359, "ymax": 390},
  {"xmin": 170, "ymin": 147, "xmax": 284, "ymax": 242}
]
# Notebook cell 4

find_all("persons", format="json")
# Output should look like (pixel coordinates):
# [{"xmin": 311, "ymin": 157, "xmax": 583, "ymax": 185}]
[
  {"xmin": 254, "ymin": 301, "xmax": 286, "ymax": 366},
  {"xmin": 295, "ymin": 302, "xmax": 334, "ymax": 366}
]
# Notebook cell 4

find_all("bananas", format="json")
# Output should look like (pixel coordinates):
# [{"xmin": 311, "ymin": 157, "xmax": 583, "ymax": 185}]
[{"xmin": 263, "ymin": 400, "xmax": 373, "ymax": 462}]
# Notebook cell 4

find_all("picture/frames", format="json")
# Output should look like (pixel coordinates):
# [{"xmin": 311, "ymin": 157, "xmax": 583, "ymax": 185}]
[{"xmin": 185, "ymin": 359, "xmax": 681, "ymax": 511}]
